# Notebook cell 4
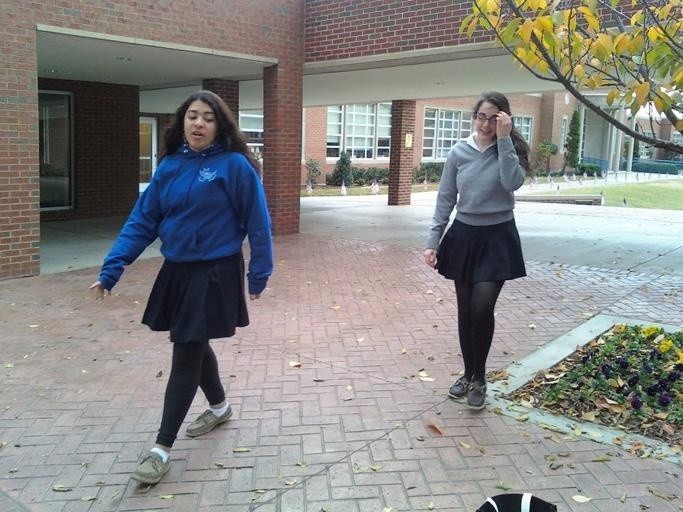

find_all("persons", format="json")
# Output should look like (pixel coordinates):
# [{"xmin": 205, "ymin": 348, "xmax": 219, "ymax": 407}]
[
  {"xmin": 421, "ymin": 89, "xmax": 536, "ymax": 412},
  {"xmin": 88, "ymin": 89, "xmax": 273, "ymax": 485}
]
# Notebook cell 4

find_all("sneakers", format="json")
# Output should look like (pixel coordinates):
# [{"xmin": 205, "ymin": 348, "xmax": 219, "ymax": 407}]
[
  {"xmin": 466, "ymin": 375, "xmax": 486, "ymax": 410},
  {"xmin": 448, "ymin": 376, "xmax": 468, "ymax": 398},
  {"xmin": 133, "ymin": 451, "xmax": 170, "ymax": 484},
  {"xmin": 187, "ymin": 405, "xmax": 233, "ymax": 436}
]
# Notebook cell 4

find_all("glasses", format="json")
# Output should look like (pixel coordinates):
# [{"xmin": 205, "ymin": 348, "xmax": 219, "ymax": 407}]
[{"xmin": 476, "ymin": 113, "xmax": 497, "ymax": 126}]
